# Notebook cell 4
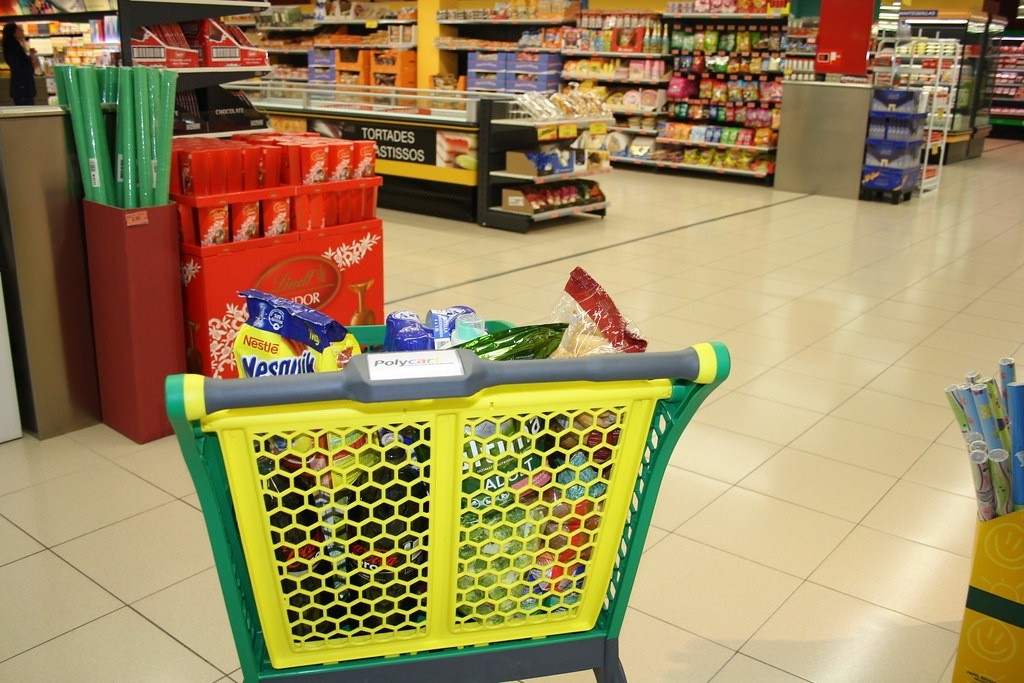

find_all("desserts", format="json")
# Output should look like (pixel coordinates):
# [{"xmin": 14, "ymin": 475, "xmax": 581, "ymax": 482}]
[{"xmin": 437, "ymin": 133, "xmax": 478, "ymax": 170}]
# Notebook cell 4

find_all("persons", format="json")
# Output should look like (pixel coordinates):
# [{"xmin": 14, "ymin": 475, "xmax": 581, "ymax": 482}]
[{"xmin": 2, "ymin": 23, "xmax": 39, "ymax": 106}]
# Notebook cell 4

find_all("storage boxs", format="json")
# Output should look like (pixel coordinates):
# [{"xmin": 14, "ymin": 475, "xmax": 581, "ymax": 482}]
[
  {"xmin": 506, "ymin": 53, "xmax": 562, "ymax": 72},
  {"xmin": 178, "ymin": 18, "xmax": 267, "ymax": 67},
  {"xmin": 507, "ymin": 150, "xmax": 577, "ymax": 176},
  {"xmin": 501, "ymin": 179, "xmax": 606, "ymax": 214},
  {"xmin": 173, "ymin": 175, "xmax": 384, "ymax": 379},
  {"xmin": 131, "ymin": 26, "xmax": 199, "ymax": 68},
  {"xmin": 467, "ymin": 52, "xmax": 516, "ymax": 72},
  {"xmin": 952, "ymin": 509, "xmax": 1024, "ymax": 683},
  {"xmin": 506, "ymin": 73, "xmax": 559, "ymax": 94},
  {"xmin": 83, "ymin": 198, "xmax": 186, "ymax": 444},
  {"xmin": 466, "ymin": 71, "xmax": 506, "ymax": 92}
]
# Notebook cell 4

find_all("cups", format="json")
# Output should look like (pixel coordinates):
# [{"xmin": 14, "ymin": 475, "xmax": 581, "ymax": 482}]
[{"xmin": 451, "ymin": 313, "xmax": 488, "ymax": 349}]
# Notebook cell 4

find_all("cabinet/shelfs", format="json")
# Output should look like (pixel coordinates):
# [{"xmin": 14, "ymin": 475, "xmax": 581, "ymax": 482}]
[
  {"xmin": 869, "ymin": 9, "xmax": 1024, "ymax": 165},
  {"xmin": 0, "ymin": 0, "xmax": 274, "ymax": 139},
  {"xmin": 223, "ymin": 0, "xmax": 820, "ymax": 178}
]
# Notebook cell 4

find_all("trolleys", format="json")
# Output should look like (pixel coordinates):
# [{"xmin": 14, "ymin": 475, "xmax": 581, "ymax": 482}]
[{"xmin": 165, "ymin": 352, "xmax": 735, "ymax": 679}]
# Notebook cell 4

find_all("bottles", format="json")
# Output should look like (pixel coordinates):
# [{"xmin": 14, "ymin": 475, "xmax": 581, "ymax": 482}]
[
  {"xmin": 642, "ymin": 24, "xmax": 651, "ymax": 53},
  {"xmin": 657, "ymin": 24, "xmax": 661, "ymax": 53},
  {"xmin": 645, "ymin": 60, "xmax": 665, "ymax": 81},
  {"xmin": 253, "ymin": 434, "xmax": 341, "ymax": 641},
  {"xmin": 347, "ymin": 434, "xmax": 428, "ymax": 635},
  {"xmin": 662, "ymin": 23, "xmax": 669, "ymax": 53},
  {"xmin": 650, "ymin": 23, "xmax": 657, "ymax": 53}
]
